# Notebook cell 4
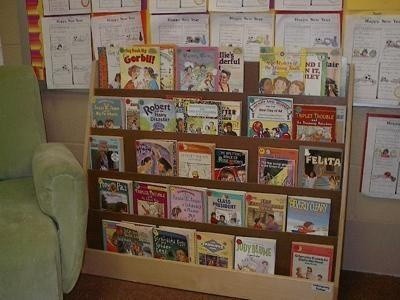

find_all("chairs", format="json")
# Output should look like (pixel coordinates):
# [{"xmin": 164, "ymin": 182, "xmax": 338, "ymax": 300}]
[{"xmin": 0, "ymin": 64, "xmax": 90, "ymax": 300}]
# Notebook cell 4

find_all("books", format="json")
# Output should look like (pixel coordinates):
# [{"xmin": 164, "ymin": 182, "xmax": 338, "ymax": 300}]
[{"xmin": 89, "ymin": 47, "xmax": 349, "ymax": 282}]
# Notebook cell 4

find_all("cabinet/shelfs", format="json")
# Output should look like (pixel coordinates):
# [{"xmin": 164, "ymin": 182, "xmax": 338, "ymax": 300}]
[{"xmin": 81, "ymin": 60, "xmax": 355, "ymax": 300}]
